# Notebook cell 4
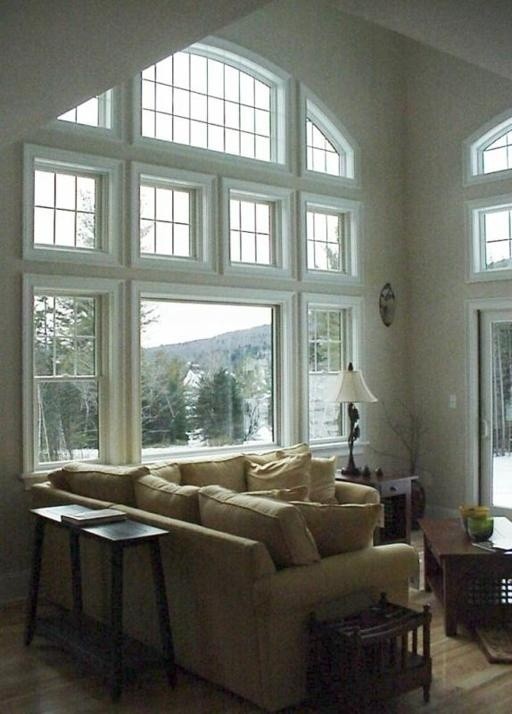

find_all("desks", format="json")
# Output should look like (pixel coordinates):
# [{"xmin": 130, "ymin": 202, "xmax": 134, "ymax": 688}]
[{"xmin": 23, "ymin": 504, "xmax": 179, "ymax": 703}]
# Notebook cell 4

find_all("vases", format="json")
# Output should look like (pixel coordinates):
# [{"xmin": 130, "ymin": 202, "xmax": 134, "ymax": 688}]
[{"xmin": 410, "ymin": 480, "xmax": 427, "ymax": 532}]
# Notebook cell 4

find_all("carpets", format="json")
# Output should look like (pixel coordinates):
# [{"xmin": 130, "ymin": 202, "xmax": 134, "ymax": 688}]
[{"xmin": 466, "ymin": 620, "xmax": 512, "ymax": 666}]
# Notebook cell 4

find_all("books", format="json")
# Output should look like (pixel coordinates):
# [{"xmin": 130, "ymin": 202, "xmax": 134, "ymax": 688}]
[{"xmin": 471, "ymin": 539, "xmax": 511, "ymax": 554}]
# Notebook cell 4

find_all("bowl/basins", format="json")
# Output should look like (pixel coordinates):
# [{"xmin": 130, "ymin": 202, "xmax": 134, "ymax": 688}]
[{"xmin": 458, "ymin": 503, "xmax": 495, "ymax": 541}]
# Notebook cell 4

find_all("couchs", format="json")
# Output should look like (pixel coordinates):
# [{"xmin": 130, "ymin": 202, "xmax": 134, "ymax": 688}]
[{"xmin": 29, "ymin": 442, "xmax": 422, "ymax": 714}]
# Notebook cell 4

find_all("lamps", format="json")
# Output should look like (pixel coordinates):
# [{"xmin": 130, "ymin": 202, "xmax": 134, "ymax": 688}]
[{"xmin": 327, "ymin": 361, "xmax": 378, "ymax": 478}]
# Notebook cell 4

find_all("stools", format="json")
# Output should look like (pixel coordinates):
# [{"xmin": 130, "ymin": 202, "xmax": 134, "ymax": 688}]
[{"xmin": 306, "ymin": 589, "xmax": 431, "ymax": 714}]
